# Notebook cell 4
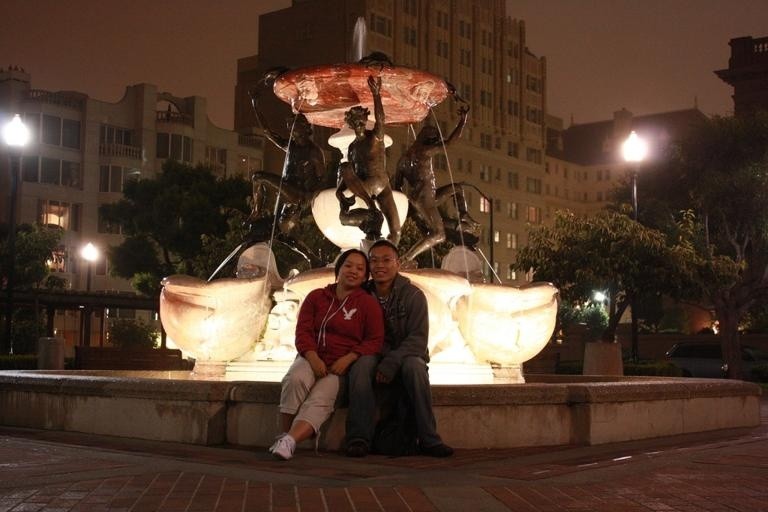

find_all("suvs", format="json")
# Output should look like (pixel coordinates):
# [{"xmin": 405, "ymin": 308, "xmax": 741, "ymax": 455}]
[{"xmin": 663, "ymin": 341, "xmax": 768, "ymax": 380}]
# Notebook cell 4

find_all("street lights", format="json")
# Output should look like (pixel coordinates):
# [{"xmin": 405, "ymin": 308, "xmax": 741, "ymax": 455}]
[
  {"xmin": 1, "ymin": 112, "xmax": 31, "ymax": 353},
  {"xmin": 619, "ymin": 129, "xmax": 648, "ymax": 365},
  {"xmin": 79, "ymin": 242, "xmax": 100, "ymax": 348}
]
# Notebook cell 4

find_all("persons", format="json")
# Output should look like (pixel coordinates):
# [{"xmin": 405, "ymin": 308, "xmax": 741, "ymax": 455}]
[
  {"xmin": 341, "ymin": 238, "xmax": 454, "ymax": 458},
  {"xmin": 267, "ymin": 248, "xmax": 386, "ymax": 461},
  {"xmin": 243, "ymin": 84, "xmax": 326, "ymax": 226},
  {"xmin": 334, "ymin": 75, "xmax": 403, "ymax": 246},
  {"xmin": 393, "ymin": 103, "xmax": 481, "ymax": 263}
]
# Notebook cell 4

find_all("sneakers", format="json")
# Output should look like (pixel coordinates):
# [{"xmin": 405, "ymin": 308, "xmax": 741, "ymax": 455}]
[
  {"xmin": 417, "ymin": 442, "xmax": 454, "ymax": 458},
  {"xmin": 345, "ymin": 440, "xmax": 368, "ymax": 458},
  {"xmin": 269, "ymin": 432, "xmax": 296, "ymax": 461}
]
